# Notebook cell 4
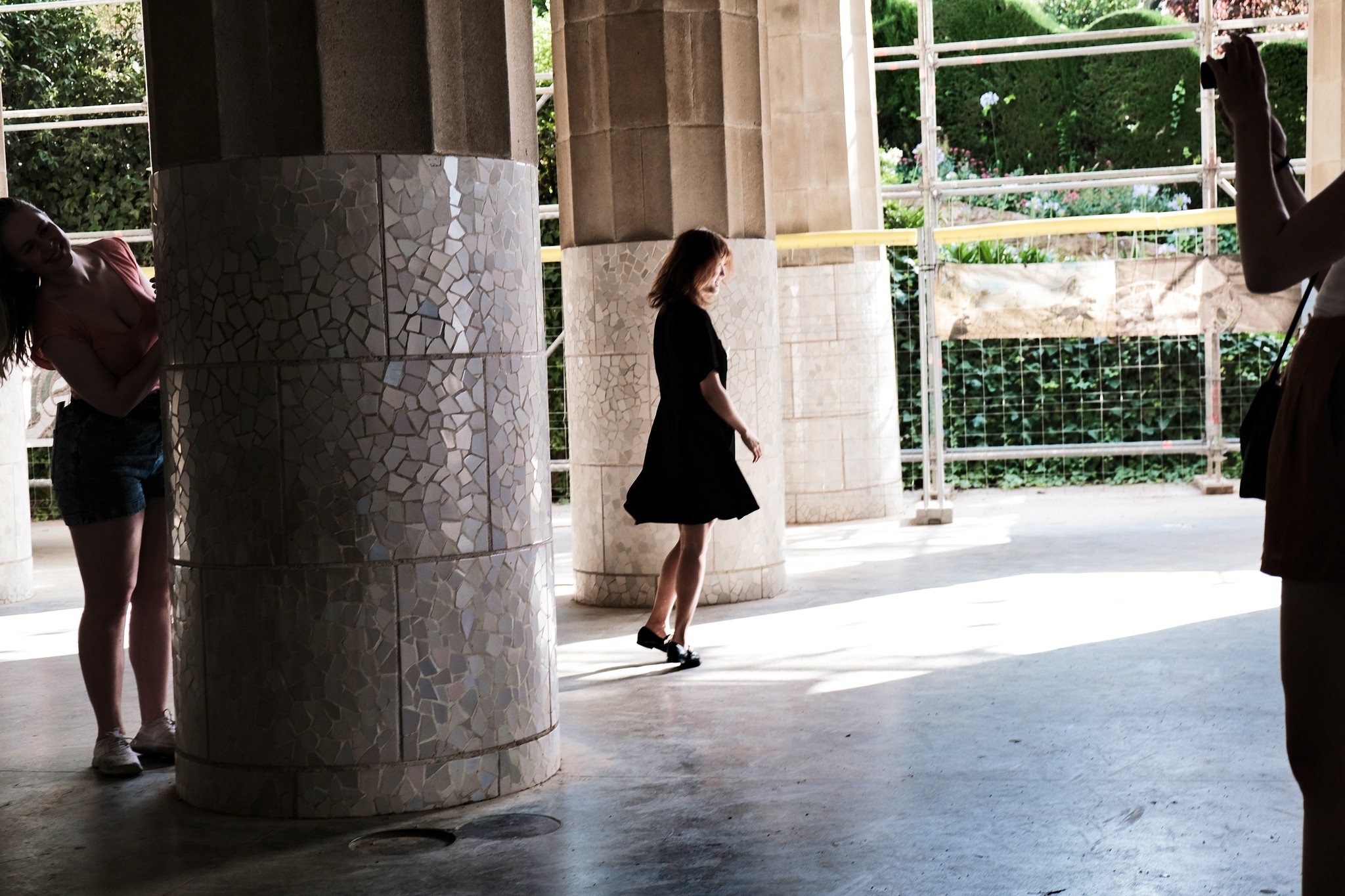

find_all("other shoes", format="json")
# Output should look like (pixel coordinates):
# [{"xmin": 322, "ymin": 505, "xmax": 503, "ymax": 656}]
[
  {"xmin": 636, "ymin": 626, "xmax": 670, "ymax": 653},
  {"xmin": 667, "ymin": 641, "xmax": 702, "ymax": 668}
]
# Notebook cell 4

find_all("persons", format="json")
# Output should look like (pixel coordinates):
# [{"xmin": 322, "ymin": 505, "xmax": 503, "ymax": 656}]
[
  {"xmin": 0, "ymin": 195, "xmax": 179, "ymax": 781},
  {"xmin": 624, "ymin": 227, "xmax": 762, "ymax": 669},
  {"xmin": 1224, "ymin": 34, "xmax": 1345, "ymax": 896}
]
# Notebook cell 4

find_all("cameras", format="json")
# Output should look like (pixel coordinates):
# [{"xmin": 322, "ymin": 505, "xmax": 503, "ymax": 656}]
[{"xmin": 1201, "ymin": 52, "xmax": 1229, "ymax": 91}]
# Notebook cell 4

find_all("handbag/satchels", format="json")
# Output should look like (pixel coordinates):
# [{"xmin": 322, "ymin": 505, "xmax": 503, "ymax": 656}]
[{"xmin": 1239, "ymin": 381, "xmax": 1283, "ymax": 501}]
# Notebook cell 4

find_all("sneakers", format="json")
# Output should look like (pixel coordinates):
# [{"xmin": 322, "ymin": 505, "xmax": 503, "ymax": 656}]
[
  {"xmin": 92, "ymin": 732, "xmax": 143, "ymax": 776},
  {"xmin": 129, "ymin": 708, "xmax": 177, "ymax": 756}
]
